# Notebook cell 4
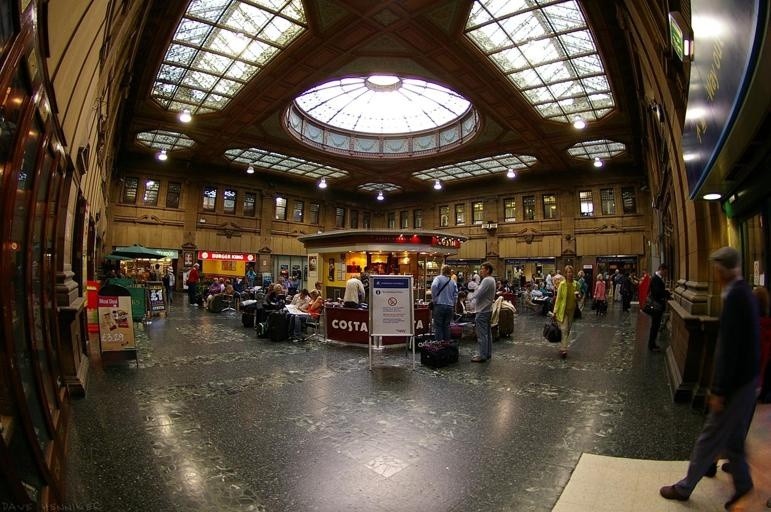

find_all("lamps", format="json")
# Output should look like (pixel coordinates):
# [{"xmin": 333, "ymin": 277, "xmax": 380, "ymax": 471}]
[
  {"xmin": 506, "ymin": 167, "xmax": 515, "ymax": 177},
  {"xmin": 246, "ymin": 162, "xmax": 254, "ymax": 173},
  {"xmin": 377, "ymin": 191, "xmax": 384, "ymax": 200},
  {"xmin": 158, "ymin": 146, "xmax": 168, "ymax": 161},
  {"xmin": 319, "ymin": 178, "xmax": 327, "ymax": 188},
  {"xmin": 434, "ymin": 180, "xmax": 441, "ymax": 189},
  {"xmin": 180, "ymin": 107, "xmax": 192, "ymax": 123},
  {"xmin": 593, "ymin": 156, "xmax": 602, "ymax": 167},
  {"xmin": 574, "ymin": 114, "xmax": 586, "ymax": 129}
]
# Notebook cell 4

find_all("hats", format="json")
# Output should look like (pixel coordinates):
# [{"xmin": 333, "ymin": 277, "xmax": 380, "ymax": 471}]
[
  {"xmin": 708, "ymin": 246, "xmax": 739, "ymax": 271},
  {"xmin": 167, "ymin": 266, "xmax": 174, "ymax": 273}
]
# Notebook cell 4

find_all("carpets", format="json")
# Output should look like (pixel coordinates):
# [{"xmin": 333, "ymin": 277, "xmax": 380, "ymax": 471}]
[{"xmin": 547, "ymin": 451, "xmax": 736, "ymax": 512}]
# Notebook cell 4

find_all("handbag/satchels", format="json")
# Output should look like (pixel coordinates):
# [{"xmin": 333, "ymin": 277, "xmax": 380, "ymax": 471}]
[
  {"xmin": 591, "ymin": 297, "xmax": 597, "ymax": 311},
  {"xmin": 573, "ymin": 303, "xmax": 583, "ymax": 322},
  {"xmin": 641, "ymin": 301, "xmax": 662, "ymax": 318},
  {"xmin": 542, "ymin": 318, "xmax": 562, "ymax": 344},
  {"xmin": 428, "ymin": 301, "xmax": 434, "ymax": 311},
  {"xmin": 150, "ymin": 272, "xmax": 157, "ymax": 281},
  {"xmin": 185, "ymin": 280, "xmax": 196, "ymax": 286},
  {"xmin": 163, "ymin": 274, "xmax": 170, "ymax": 287},
  {"xmin": 620, "ymin": 286, "xmax": 631, "ymax": 297}
]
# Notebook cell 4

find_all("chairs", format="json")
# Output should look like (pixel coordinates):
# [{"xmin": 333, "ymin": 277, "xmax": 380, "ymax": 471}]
[
  {"xmin": 305, "ymin": 307, "xmax": 324, "ymax": 341},
  {"xmin": 221, "ymin": 294, "xmax": 236, "ymax": 312}
]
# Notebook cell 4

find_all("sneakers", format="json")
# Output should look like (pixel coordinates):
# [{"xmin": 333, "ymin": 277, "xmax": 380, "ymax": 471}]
[
  {"xmin": 703, "ymin": 461, "xmax": 721, "ymax": 478},
  {"xmin": 189, "ymin": 303, "xmax": 199, "ymax": 307},
  {"xmin": 659, "ymin": 485, "xmax": 690, "ymax": 502},
  {"xmin": 725, "ymin": 488, "xmax": 754, "ymax": 510},
  {"xmin": 721, "ymin": 462, "xmax": 733, "ymax": 473}
]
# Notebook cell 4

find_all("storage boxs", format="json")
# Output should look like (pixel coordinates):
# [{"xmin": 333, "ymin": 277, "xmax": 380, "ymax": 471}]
[{"xmin": 422, "ymin": 343, "xmax": 459, "ymax": 368}]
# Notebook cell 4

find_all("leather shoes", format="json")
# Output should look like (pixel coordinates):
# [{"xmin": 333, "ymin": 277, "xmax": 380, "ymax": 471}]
[
  {"xmin": 559, "ymin": 351, "xmax": 567, "ymax": 359},
  {"xmin": 470, "ymin": 355, "xmax": 488, "ymax": 362},
  {"xmin": 650, "ymin": 345, "xmax": 661, "ymax": 352}
]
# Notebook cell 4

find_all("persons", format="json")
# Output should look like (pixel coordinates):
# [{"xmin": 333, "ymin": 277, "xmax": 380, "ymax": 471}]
[
  {"xmin": 145, "ymin": 266, "xmax": 154, "ymax": 273},
  {"xmin": 659, "ymin": 246, "xmax": 764, "ymax": 512},
  {"xmin": 185, "ymin": 262, "xmax": 200, "ymax": 307},
  {"xmin": 165, "ymin": 266, "xmax": 176, "ymax": 304},
  {"xmin": 451, "ymin": 266, "xmax": 650, "ymax": 322},
  {"xmin": 152, "ymin": 264, "xmax": 161, "ymax": 282},
  {"xmin": 702, "ymin": 283, "xmax": 770, "ymax": 479},
  {"xmin": 203, "ymin": 266, "xmax": 325, "ymax": 343},
  {"xmin": 343, "ymin": 274, "xmax": 366, "ymax": 308},
  {"xmin": 429, "ymin": 265, "xmax": 458, "ymax": 343},
  {"xmin": 647, "ymin": 263, "xmax": 674, "ymax": 352},
  {"xmin": 550, "ymin": 264, "xmax": 583, "ymax": 357},
  {"xmin": 469, "ymin": 262, "xmax": 497, "ymax": 362}
]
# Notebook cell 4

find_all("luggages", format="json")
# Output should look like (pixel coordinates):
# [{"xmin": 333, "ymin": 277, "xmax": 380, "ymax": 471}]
[
  {"xmin": 602, "ymin": 300, "xmax": 608, "ymax": 315},
  {"xmin": 419, "ymin": 339, "xmax": 460, "ymax": 371}
]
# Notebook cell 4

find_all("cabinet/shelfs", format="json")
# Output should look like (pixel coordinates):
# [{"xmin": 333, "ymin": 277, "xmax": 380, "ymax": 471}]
[{"xmin": 0, "ymin": 0, "xmax": 74, "ymax": 512}]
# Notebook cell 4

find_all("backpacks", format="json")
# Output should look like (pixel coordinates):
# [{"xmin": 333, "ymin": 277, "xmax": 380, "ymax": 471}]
[{"xmin": 255, "ymin": 320, "xmax": 269, "ymax": 339}]
